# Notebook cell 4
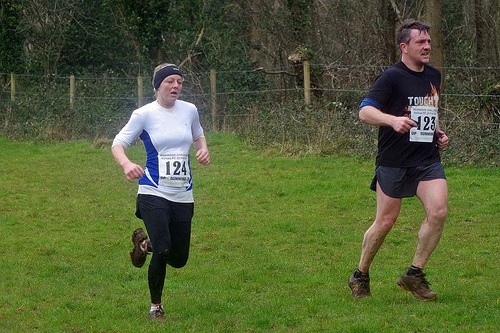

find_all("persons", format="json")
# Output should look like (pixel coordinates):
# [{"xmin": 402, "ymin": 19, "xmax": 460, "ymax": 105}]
[
  {"xmin": 347, "ymin": 19, "xmax": 449, "ymax": 301},
  {"xmin": 110, "ymin": 62, "xmax": 211, "ymax": 321}
]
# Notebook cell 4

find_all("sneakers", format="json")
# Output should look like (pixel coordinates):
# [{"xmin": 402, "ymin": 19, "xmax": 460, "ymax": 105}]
[
  {"xmin": 130, "ymin": 228, "xmax": 148, "ymax": 268},
  {"xmin": 397, "ymin": 268, "xmax": 437, "ymax": 301},
  {"xmin": 149, "ymin": 309, "xmax": 166, "ymax": 320},
  {"xmin": 348, "ymin": 268, "xmax": 372, "ymax": 297}
]
